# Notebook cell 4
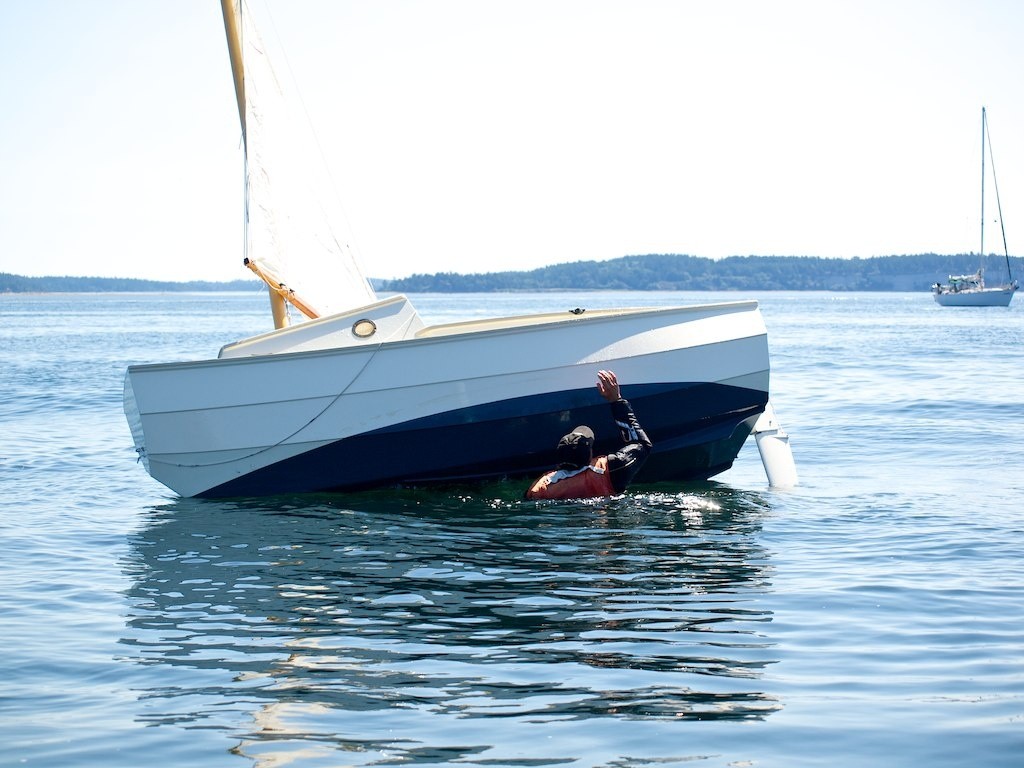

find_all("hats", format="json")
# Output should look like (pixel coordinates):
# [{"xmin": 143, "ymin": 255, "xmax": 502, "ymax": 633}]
[{"xmin": 557, "ymin": 424, "xmax": 595, "ymax": 467}]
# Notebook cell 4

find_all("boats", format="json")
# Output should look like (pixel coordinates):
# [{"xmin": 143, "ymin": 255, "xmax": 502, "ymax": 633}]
[
  {"xmin": 123, "ymin": 0, "xmax": 773, "ymax": 501},
  {"xmin": 933, "ymin": 106, "xmax": 1021, "ymax": 306}
]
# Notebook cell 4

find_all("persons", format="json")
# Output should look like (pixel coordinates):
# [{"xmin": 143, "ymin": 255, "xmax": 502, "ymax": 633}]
[{"xmin": 523, "ymin": 369, "xmax": 654, "ymax": 506}]
